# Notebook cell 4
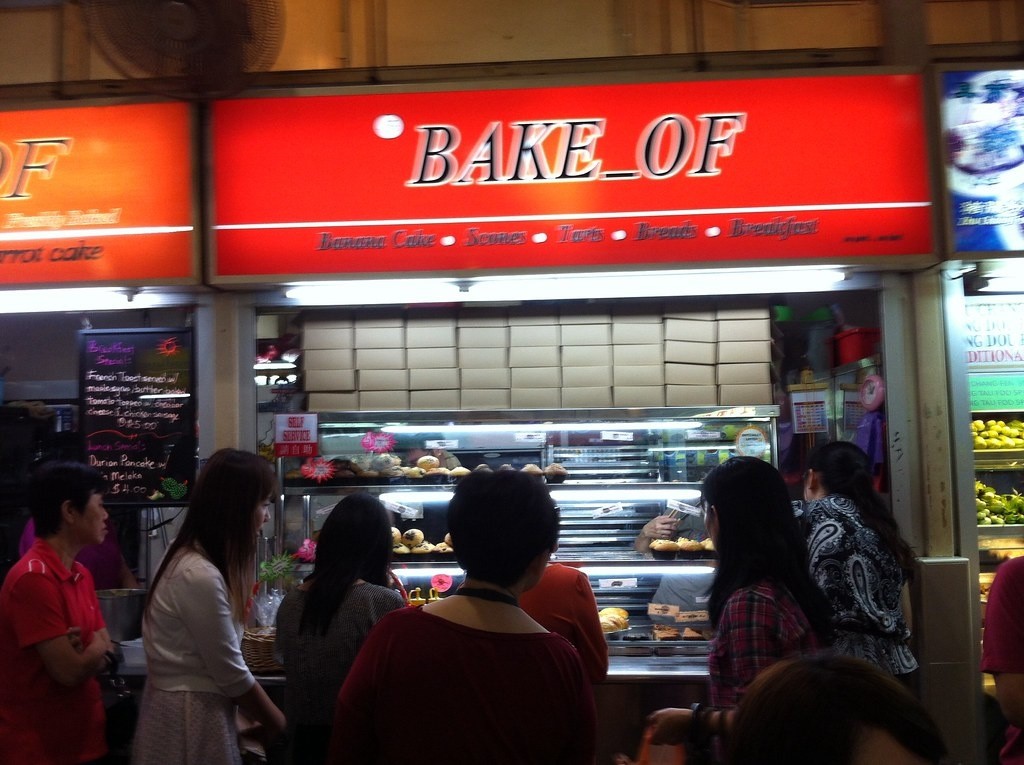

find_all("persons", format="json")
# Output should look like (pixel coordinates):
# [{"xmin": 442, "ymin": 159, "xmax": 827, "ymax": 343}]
[
  {"xmin": 326, "ymin": 470, "xmax": 599, "ymax": 765},
  {"xmin": 632, "ymin": 441, "xmax": 1024, "ymax": 765},
  {"xmin": 0, "ymin": 438, "xmax": 138, "ymax": 765},
  {"xmin": 274, "ymin": 493, "xmax": 406, "ymax": 765},
  {"xmin": 127, "ymin": 446, "xmax": 288, "ymax": 764}
]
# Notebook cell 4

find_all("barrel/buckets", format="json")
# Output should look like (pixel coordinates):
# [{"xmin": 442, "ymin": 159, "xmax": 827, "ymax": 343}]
[
  {"xmin": 93, "ymin": 588, "xmax": 148, "ymax": 658},
  {"xmin": 833, "ymin": 325, "xmax": 881, "ymax": 365}
]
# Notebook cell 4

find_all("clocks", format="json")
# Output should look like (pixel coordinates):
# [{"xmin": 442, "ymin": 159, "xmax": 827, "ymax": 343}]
[{"xmin": 861, "ymin": 374, "xmax": 885, "ymax": 411}]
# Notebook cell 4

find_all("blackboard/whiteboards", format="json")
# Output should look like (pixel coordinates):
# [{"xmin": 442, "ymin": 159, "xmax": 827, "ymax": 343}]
[{"xmin": 76, "ymin": 325, "xmax": 197, "ymax": 506}]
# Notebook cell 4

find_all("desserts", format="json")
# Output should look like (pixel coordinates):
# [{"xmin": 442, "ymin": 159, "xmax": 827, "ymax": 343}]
[{"xmin": 319, "ymin": 450, "xmax": 718, "ymax": 643}]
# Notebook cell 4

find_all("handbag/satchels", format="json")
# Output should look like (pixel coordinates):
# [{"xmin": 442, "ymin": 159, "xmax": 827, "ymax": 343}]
[
  {"xmin": 94, "ymin": 649, "xmax": 139, "ymax": 754},
  {"xmin": 633, "ymin": 721, "xmax": 686, "ymax": 765}
]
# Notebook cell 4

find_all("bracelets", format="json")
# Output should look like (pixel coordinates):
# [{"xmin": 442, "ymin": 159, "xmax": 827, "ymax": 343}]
[{"xmin": 700, "ymin": 703, "xmax": 734, "ymax": 740}]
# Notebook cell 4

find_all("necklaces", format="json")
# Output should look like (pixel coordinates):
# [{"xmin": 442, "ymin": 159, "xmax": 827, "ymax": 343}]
[{"xmin": 456, "ymin": 588, "xmax": 519, "ymax": 608}]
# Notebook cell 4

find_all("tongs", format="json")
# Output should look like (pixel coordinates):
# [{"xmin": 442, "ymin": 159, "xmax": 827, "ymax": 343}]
[{"xmin": 650, "ymin": 501, "xmax": 704, "ymax": 542}]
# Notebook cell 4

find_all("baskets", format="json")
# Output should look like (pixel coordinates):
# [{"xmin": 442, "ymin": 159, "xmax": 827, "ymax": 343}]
[{"xmin": 240, "ymin": 551, "xmax": 316, "ymax": 672}]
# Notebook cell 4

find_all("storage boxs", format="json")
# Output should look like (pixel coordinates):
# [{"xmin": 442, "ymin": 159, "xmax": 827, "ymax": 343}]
[
  {"xmin": 357, "ymin": 369, "xmax": 410, "ymax": 391},
  {"xmin": 303, "ymin": 350, "xmax": 354, "ymax": 369},
  {"xmin": 354, "ymin": 307, "xmax": 406, "ymax": 328},
  {"xmin": 405, "ymin": 327, "xmax": 457, "ymax": 349},
  {"xmin": 410, "ymin": 369, "xmax": 461, "ymax": 391},
  {"xmin": 308, "ymin": 391, "xmax": 360, "ymax": 411},
  {"xmin": 359, "ymin": 391, "xmax": 409, "ymax": 410},
  {"xmin": 305, "ymin": 370, "xmax": 356, "ymax": 391},
  {"xmin": 355, "ymin": 349, "xmax": 407, "ymax": 370},
  {"xmin": 406, "ymin": 306, "xmax": 457, "ymax": 327},
  {"xmin": 407, "ymin": 348, "xmax": 458, "ymax": 369},
  {"xmin": 409, "ymin": 391, "xmax": 461, "ymax": 410},
  {"xmin": 304, "ymin": 307, "xmax": 353, "ymax": 328},
  {"xmin": 302, "ymin": 327, "xmax": 354, "ymax": 350},
  {"xmin": 459, "ymin": 308, "xmax": 772, "ymax": 411},
  {"xmin": 835, "ymin": 327, "xmax": 881, "ymax": 364},
  {"xmin": 353, "ymin": 327, "xmax": 405, "ymax": 348}
]
